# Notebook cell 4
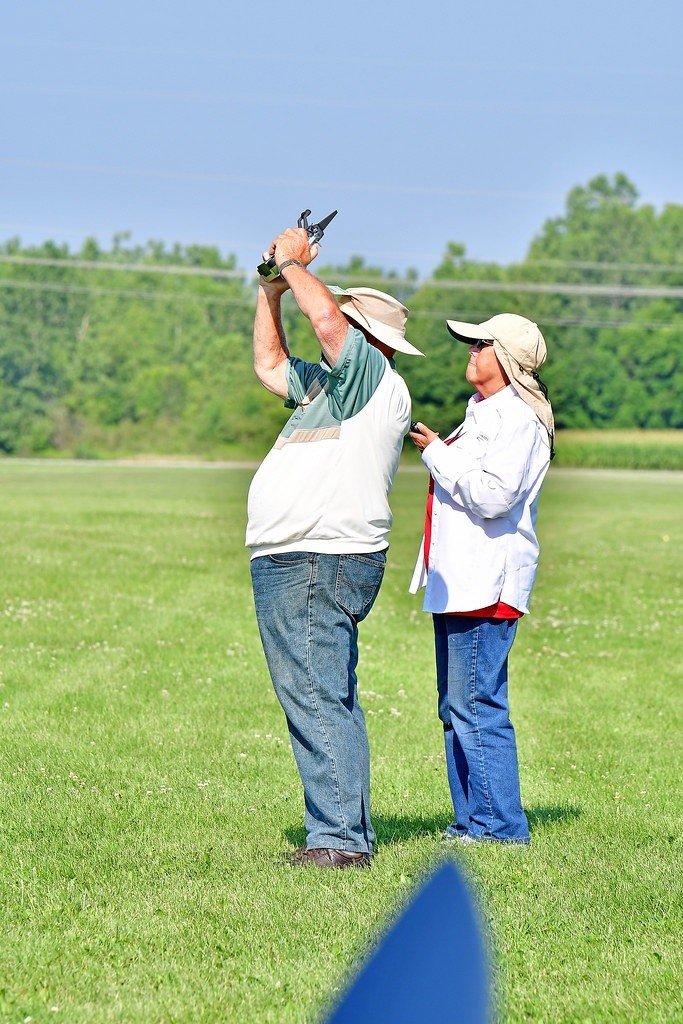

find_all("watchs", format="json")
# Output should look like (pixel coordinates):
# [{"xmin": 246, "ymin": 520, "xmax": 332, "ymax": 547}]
[{"xmin": 278, "ymin": 259, "xmax": 303, "ymax": 281}]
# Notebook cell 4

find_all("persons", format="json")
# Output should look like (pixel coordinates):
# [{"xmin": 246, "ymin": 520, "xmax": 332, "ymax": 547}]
[
  {"xmin": 407, "ymin": 313, "xmax": 554, "ymax": 846},
  {"xmin": 245, "ymin": 227, "xmax": 426, "ymax": 869}
]
